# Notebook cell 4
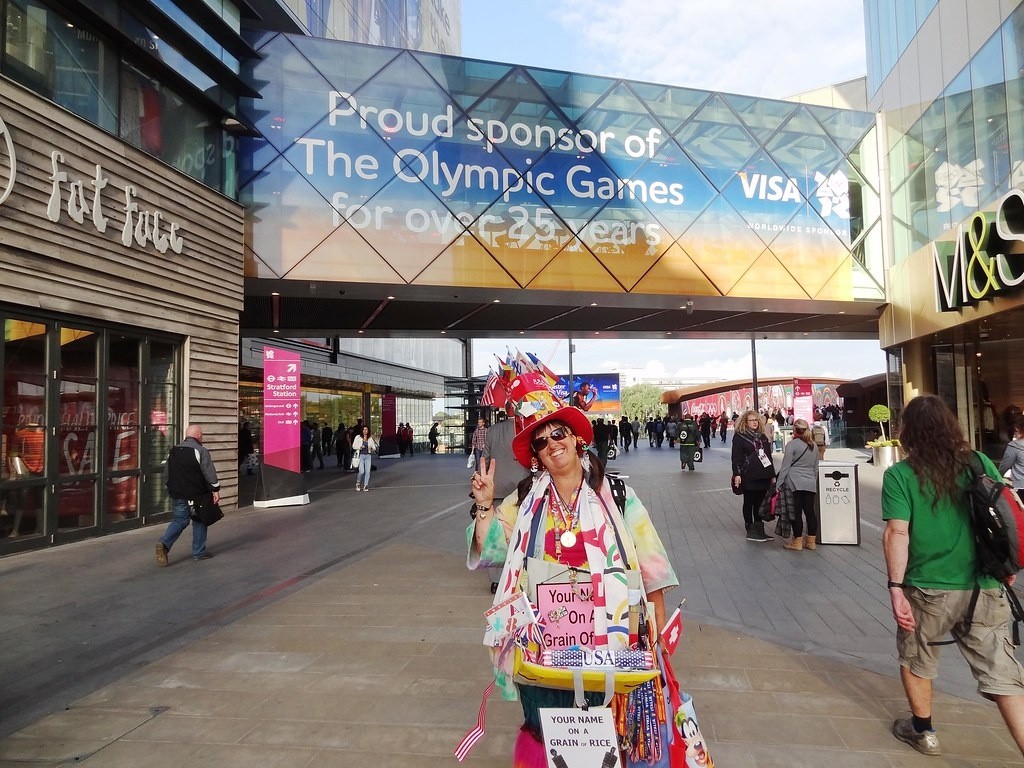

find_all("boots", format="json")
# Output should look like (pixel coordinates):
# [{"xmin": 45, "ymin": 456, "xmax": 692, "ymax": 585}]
[
  {"xmin": 803, "ymin": 535, "xmax": 816, "ymax": 550},
  {"xmin": 745, "ymin": 523, "xmax": 767, "ymax": 541},
  {"xmin": 783, "ymin": 536, "xmax": 802, "ymax": 550},
  {"xmin": 755, "ymin": 521, "xmax": 774, "ymax": 540}
]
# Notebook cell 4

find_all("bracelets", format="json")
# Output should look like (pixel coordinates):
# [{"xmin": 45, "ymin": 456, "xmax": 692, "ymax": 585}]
[
  {"xmin": 476, "ymin": 503, "xmax": 494, "ymax": 518},
  {"xmin": 888, "ymin": 581, "xmax": 904, "ymax": 589}
]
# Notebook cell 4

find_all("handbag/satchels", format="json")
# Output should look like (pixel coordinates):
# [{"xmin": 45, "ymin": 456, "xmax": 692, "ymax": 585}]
[
  {"xmin": 771, "ymin": 491, "xmax": 779, "ymax": 515},
  {"xmin": 655, "ymin": 645, "xmax": 714, "ymax": 768},
  {"xmin": 467, "ymin": 446, "xmax": 475, "ymax": 469},
  {"xmin": 614, "ymin": 443, "xmax": 619, "ymax": 456},
  {"xmin": 352, "ymin": 458, "xmax": 360, "ymax": 468},
  {"xmin": 628, "ymin": 644, "xmax": 670, "ymax": 768},
  {"xmin": 607, "ymin": 446, "xmax": 616, "ymax": 460},
  {"xmin": 693, "ymin": 446, "xmax": 702, "ymax": 462},
  {"xmin": 188, "ymin": 492, "xmax": 224, "ymax": 526},
  {"xmin": 397, "ymin": 433, "xmax": 402, "ymax": 445}
]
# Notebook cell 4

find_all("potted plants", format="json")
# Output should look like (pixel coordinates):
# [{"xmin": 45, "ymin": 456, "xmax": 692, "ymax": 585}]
[{"xmin": 865, "ymin": 404, "xmax": 903, "ymax": 470}]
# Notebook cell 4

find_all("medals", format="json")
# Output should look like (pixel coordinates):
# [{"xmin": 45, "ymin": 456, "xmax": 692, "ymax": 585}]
[{"xmin": 560, "ymin": 531, "xmax": 576, "ymax": 548}]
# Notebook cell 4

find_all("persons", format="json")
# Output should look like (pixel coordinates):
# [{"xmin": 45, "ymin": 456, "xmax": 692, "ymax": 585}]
[
  {"xmin": 881, "ymin": 396, "xmax": 1024, "ymax": 759},
  {"xmin": 155, "ymin": 425, "xmax": 220, "ymax": 566},
  {"xmin": 397, "ymin": 422, "xmax": 413, "ymax": 457},
  {"xmin": 761, "ymin": 409, "xmax": 794, "ymax": 453},
  {"xmin": 813, "ymin": 404, "xmax": 841, "ymax": 420},
  {"xmin": 352, "ymin": 425, "xmax": 376, "ymax": 492},
  {"xmin": 466, "ymin": 390, "xmax": 679, "ymax": 768},
  {"xmin": 679, "ymin": 413, "xmax": 700, "ymax": 471},
  {"xmin": 731, "ymin": 410, "xmax": 776, "ymax": 542},
  {"xmin": 8, "ymin": 423, "xmax": 45, "ymax": 539},
  {"xmin": 472, "ymin": 418, "xmax": 488, "ymax": 471},
  {"xmin": 997, "ymin": 415, "xmax": 1024, "ymax": 504},
  {"xmin": 300, "ymin": 419, "xmax": 363, "ymax": 472},
  {"xmin": 565, "ymin": 382, "xmax": 597, "ymax": 411},
  {"xmin": 693, "ymin": 411, "xmax": 739, "ymax": 448},
  {"xmin": 238, "ymin": 422, "xmax": 255, "ymax": 476},
  {"xmin": 430, "ymin": 423, "xmax": 439, "ymax": 454},
  {"xmin": 590, "ymin": 415, "xmax": 684, "ymax": 466},
  {"xmin": 776, "ymin": 419, "xmax": 819, "ymax": 550},
  {"xmin": 482, "ymin": 418, "xmax": 531, "ymax": 594}
]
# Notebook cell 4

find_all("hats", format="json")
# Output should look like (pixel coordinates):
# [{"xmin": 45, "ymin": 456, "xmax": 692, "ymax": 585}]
[{"xmin": 503, "ymin": 373, "xmax": 594, "ymax": 471}]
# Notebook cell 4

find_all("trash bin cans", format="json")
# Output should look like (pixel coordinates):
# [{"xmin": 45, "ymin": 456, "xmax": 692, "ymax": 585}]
[
  {"xmin": 778, "ymin": 426, "xmax": 793, "ymax": 454},
  {"xmin": 814, "ymin": 460, "xmax": 861, "ymax": 545}
]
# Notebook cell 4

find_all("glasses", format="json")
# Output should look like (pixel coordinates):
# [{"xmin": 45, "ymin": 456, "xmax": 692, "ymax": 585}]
[
  {"xmin": 747, "ymin": 419, "xmax": 758, "ymax": 421},
  {"xmin": 531, "ymin": 426, "xmax": 572, "ymax": 452}
]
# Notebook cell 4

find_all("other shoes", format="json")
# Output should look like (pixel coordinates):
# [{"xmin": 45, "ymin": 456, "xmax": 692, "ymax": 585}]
[
  {"xmin": 193, "ymin": 551, "xmax": 214, "ymax": 560},
  {"xmin": 731, "ymin": 471, "xmax": 743, "ymax": 495},
  {"xmin": 621, "ymin": 435, "xmax": 726, "ymax": 471},
  {"xmin": 491, "ymin": 582, "xmax": 498, "ymax": 594},
  {"xmin": 156, "ymin": 542, "xmax": 169, "ymax": 567},
  {"xmin": 301, "ymin": 462, "xmax": 369, "ymax": 491},
  {"xmin": 893, "ymin": 710, "xmax": 942, "ymax": 756}
]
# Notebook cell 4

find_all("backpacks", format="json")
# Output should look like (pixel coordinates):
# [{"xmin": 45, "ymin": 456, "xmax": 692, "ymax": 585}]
[
  {"xmin": 811, "ymin": 423, "xmax": 825, "ymax": 446},
  {"xmin": 656, "ymin": 423, "xmax": 663, "ymax": 432},
  {"xmin": 928, "ymin": 449, "xmax": 1024, "ymax": 581}
]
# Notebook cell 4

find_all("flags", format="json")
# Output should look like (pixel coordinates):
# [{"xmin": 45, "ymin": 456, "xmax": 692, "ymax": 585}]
[
  {"xmin": 662, "ymin": 608, "xmax": 682, "ymax": 656},
  {"xmin": 515, "ymin": 597, "xmax": 547, "ymax": 644},
  {"xmin": 480, "ymin": 351, "xmax": 560, "ymax": 408}
]
